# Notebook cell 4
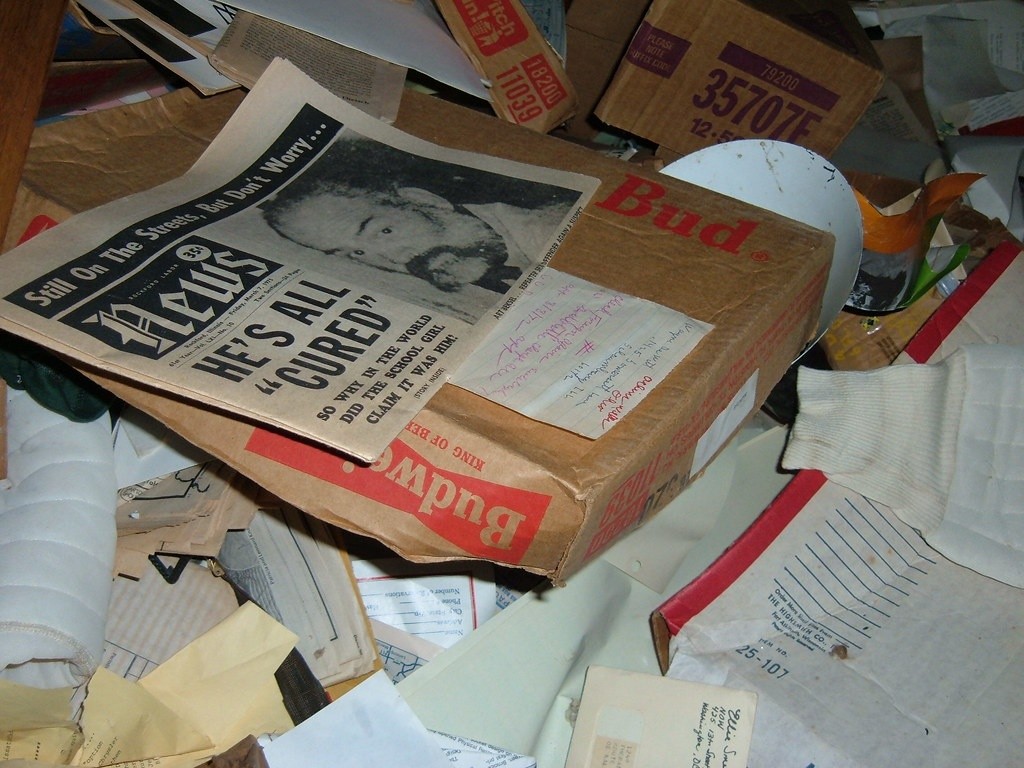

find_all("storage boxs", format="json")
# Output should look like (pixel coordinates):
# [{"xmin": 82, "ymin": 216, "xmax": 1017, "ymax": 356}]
[{"xmin": 1, "ymin": 0, "xmax": 947, "ymax": 587}]
